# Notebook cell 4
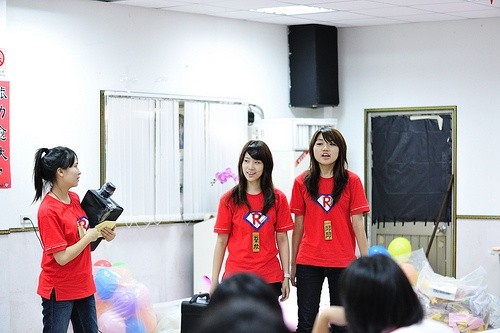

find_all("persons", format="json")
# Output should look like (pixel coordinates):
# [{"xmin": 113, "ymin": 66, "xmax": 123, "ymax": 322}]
[
  {"xmin": 209, "ymin": 140, "xmax": 295, "ymax": 302},
  {"xmin": 289, "ymin": 128, "xmax": 370, "ymax": 333},
  {"xmin": 32, "ymin": 146, "xmax": 116, "ymax": 333},
  {"xmin": 312, "ymin": 255, "xmax": 455, "ymax": 333},
  {"xmin": 195, "ymin": 274, "xmax": 294, "ymax": 333}
]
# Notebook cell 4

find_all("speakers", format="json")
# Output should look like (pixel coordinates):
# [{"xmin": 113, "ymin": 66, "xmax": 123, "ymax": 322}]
[{"xmin": 287, "ymin": 23, "xmax": 339, "ymax": 109}]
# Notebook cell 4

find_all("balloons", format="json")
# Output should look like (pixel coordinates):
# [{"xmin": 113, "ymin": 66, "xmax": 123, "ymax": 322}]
[
  {"xmin": 400, "ymin": 263, "xmax": 416, "ymax": 285},
  {"xmin": 387, "ymin": 238, "xmax": 412, "ymax": 263},
  {"xmin": 366, "ymin": 245, "xmax": 390, "ymax": 257}
]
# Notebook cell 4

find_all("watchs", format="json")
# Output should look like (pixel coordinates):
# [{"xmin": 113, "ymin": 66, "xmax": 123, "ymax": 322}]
[{"xmin": 284, "ymin": 272, "xmax": 293, "ymax": 279}]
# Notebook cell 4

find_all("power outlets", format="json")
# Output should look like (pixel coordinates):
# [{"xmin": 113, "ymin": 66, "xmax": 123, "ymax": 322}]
[{"xmin": 20, "ymin": 215, "xmax": 32, "ymax": 224}]
